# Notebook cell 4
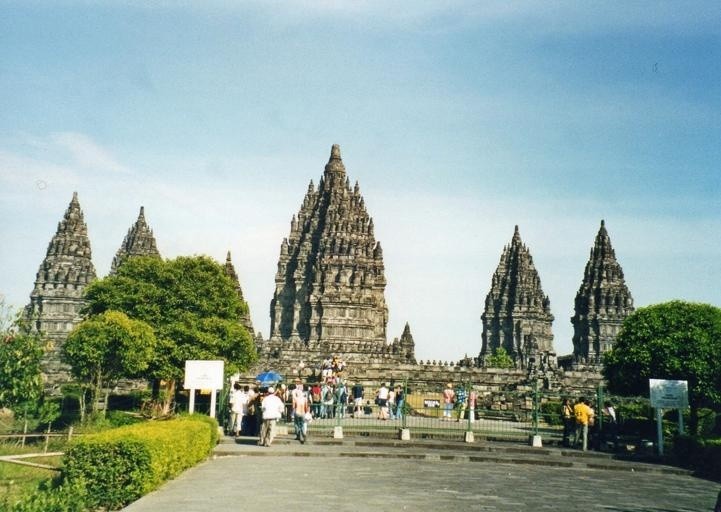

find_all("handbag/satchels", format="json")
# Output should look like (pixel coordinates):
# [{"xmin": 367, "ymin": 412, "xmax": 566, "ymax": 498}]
[
  {"xmin": 313, "ymin": 392, "xmax": 320, "ymax": 403},
  {"xmin": 375, "ymin": 398, "xmax": 382, "ymax": 404}
]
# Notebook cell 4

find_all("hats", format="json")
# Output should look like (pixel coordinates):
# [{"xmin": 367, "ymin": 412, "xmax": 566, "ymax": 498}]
[{"xmin": 294, "ymin": 378, "xmax": 302, "ymax": 381}]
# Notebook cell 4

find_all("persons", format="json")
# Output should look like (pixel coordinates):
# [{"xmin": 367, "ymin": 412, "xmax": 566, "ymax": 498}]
[
  {"xmin": 227, "ymin": 353, "xmax": 409, "ymax": 449},
  {"xmin": 573, "ymin": 394, "xmax": 593, "ymax": 453},
  {"xmin": 561, "ymin": 397, "xmax": 575, "ymax": 449},
  {"xmin": 455, "ymin": 382, "xmax": 467, "ymax": 422},
  {"xmin": 441, "ymin": 382, "xmax": 456, "ymax": 420},
  {"xmin": 600, "ymin": 401, "xmax": 623, "ymax": 453},
  {"xmin": 469, "ymin": 385, "xmax": 478, "ymax": 422}
]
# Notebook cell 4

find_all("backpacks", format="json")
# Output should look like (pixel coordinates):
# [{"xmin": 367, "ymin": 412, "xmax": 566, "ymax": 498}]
[
  {"xmin": 325, "ymin": 386, "xmax": 332, "ymax": 400},
  {"xmin": 341, "ymin": 391, "xmax": 346, "ymax": 402}
]
